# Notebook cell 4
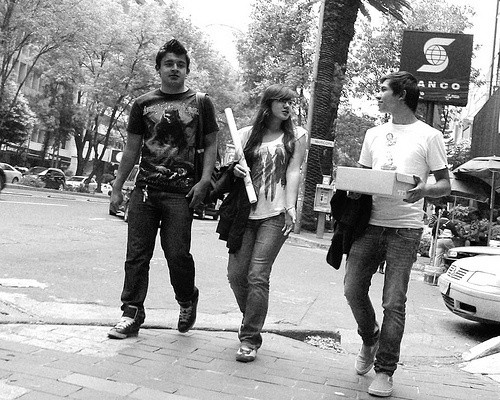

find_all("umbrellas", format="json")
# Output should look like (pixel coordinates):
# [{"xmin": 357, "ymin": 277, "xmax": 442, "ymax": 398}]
[
  {"xmin": 448, "ymin": 172, "xmax": 489, "ymax": 223},
  {"xmin": 452, "ymin": 156, "xmax": 500, "ymax": 239}
]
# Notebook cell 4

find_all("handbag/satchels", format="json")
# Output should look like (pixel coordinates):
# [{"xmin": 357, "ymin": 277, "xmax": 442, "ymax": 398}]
[{"xmin": 438, "ymin": 224, "xmax": 446, "ymax": 234}]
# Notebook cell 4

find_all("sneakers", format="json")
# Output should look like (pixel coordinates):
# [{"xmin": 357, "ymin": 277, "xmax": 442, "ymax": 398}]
[
  {"xmin": 368, "ymin": 372, "xmax": 393, "ymax": 396},
  {"xmin": 235, "ymin": 318, "xmax": 257, "ymax": 363},
  {"xmin": 178, "ymin": 289, "xmax": 199, "ymax": 333},
  {"xmin": 355, "ymin": 339, "xmax": 380, "ymax": 375},
  {"xmin": 108, "ymin": 305, "xmax": 145, "ymax": 339}
]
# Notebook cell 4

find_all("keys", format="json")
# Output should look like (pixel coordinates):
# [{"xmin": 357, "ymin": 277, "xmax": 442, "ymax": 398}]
[{"xmin": 142, "ymin": 189, "xmax": 148, "ymax": 202}]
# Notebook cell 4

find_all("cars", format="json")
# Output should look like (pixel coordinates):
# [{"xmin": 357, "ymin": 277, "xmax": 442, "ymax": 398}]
[
  {"xmin": 0, "ymin": 162, "xmax": 112, "ymax": 196},
  {"xmin": 110, "ymin": 164, "xmax": 141, "ymax": 222},
  {"xmin": 436, "ymin": 244, "xmax": 500, "ymax": 323}
]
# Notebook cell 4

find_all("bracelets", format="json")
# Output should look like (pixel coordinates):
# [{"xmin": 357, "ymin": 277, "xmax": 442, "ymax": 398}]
[{"xmin": 285, "ymin": 206, "xmax": 295, "ymax": 212}]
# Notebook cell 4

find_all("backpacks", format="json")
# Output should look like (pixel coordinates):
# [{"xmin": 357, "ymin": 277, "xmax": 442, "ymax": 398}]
[{"xmin": 195, "ymin": 91, "xmax": 223, "ymax": 216}]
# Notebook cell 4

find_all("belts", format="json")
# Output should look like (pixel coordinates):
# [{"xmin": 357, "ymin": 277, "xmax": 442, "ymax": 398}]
[{"xmin": 437, "ymin": 236, "xmax": 453, "ymax": 239}]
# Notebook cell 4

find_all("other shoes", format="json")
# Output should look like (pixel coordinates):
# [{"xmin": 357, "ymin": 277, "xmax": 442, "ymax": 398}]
[{"xmin": 378, "ymin": 269, "xmax": 384, "ymax": 274}]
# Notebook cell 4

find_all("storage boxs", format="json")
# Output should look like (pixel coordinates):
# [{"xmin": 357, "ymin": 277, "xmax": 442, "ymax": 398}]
[{"xmin": 335, "ymin": 166, "xmax": 415, "ymax": 200}]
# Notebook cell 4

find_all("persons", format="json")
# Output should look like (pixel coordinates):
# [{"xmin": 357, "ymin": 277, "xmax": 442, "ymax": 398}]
[
  {"xmin": 471, "ymin": 210, "xmax": 487, "ymax": 246},
  {"xmin": 109, "ymin": 39, "xmax": 221, "ymax": 339},
  {"xmin": 429, "ymin": 206, "xmax": 442, "ymax": 257},
  {"xmin": 432, "ymin": 212, "xmax": 457, "ymax": 267},
  {"xmin": 344, "ymin": 71, "xmax": 450, "ymax": 396},
  {"xmin": 227, "ymin": 85, "xmax": 308, "ymax": 362}
]
